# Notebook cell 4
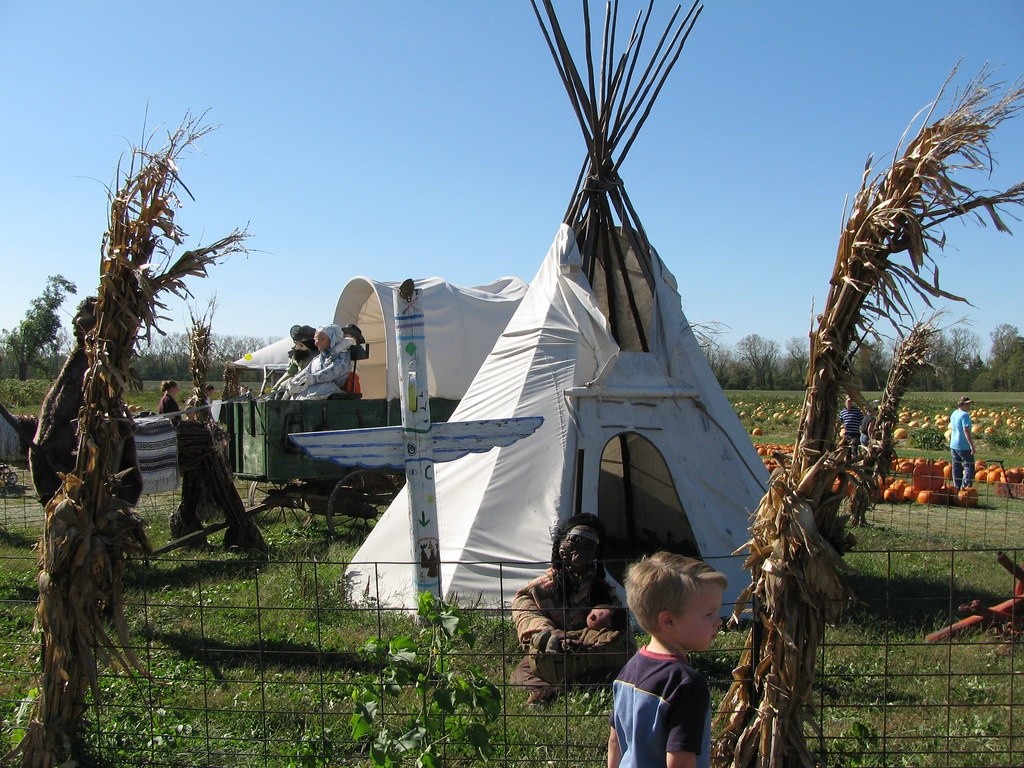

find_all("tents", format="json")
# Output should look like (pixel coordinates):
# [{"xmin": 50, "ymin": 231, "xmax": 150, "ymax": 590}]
[
  {"xmin": 233, "ymin": 325, "xmax": 326, "ymax": 398},
  {"xmin": 331, "ymin": 221, "xmax": 863, "ymax": 624}
]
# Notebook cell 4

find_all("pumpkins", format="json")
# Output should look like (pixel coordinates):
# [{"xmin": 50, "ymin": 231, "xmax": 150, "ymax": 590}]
[
  {"xmin": 752, "ymin": 441, "xmax": 795, "ymax": 473},
  {"xmin": 893, "ymin": 406, "xmax": 1024, "ymax": 442},
  {"xmin": 734, "ymin": 401, "xmax": 801, "ymax": 436},
  {"xmin": 124, "ymin": 403, "xmax": 143, "ymax": 412},
  {"xmin": 826, "ymin": 457, "xmax": 1024, "ymax": 507}
]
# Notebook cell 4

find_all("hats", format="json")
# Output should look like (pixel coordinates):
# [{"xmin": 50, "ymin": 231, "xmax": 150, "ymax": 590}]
[
  {"xmin": 958, "ymin": 395, "xmax": 974, "ymax": 405},
  {"xmin": 867, "ymin": 400, "xmax": 881, "ymax": 415}
]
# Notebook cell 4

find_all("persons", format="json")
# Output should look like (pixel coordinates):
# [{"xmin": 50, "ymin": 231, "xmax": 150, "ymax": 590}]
[
  {"xmin": 606, "ymin": 548, "xmax": 728, "ymax": 768},
  {"xmin": 203, "ymin": 384, "xmax": 215, "ymax": 424},
  {"xmin": 949, "ymin": 396, "xmax": 978, "ymax": 490},
  {"xmin": 839, "ymin": 397, "xmax": 881, "ymax": 456},
  {"xmin": 157, "ymin": 380, "xmax": 182, "ymax": 427},
  {"xmin": 267, "ymin": 319, "xmax": 357, "ymax": 402},
  {"xmin": 239, "ymin": 383, "xmax": 254, "ymax": 401},
  {"xmin": 185, "ymin": 406, "xmax": 198, "ymax": 421},
  {"xmin": 510, "ymin": 512, "xmax": 637, "ymax": 713}
]
